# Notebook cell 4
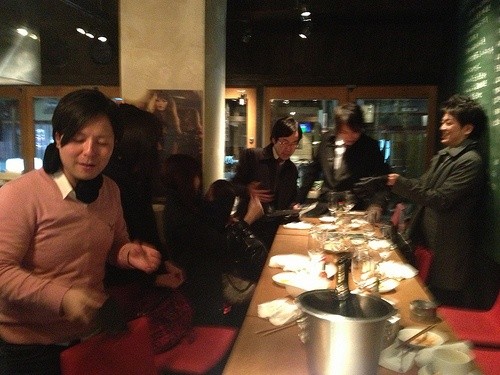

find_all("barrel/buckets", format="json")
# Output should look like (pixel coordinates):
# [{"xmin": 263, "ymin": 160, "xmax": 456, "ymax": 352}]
[{"xmin": 292, "ymin": 289, "xmax": 397, "ymax": 375}]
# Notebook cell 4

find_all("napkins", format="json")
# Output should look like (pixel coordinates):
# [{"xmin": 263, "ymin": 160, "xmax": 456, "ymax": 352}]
[
  {"xmin": 257, "ymin": 299, "xmax": 303, "ymax": 326},
  {"xmin": 270, "ymin": 254, "xmax": 310, "ymax": 271}
]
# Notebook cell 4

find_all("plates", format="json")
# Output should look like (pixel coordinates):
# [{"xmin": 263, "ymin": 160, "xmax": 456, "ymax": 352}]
[
  {"xmin": 397, "ymin": 328, "xmax": 444, "ymax": 351},
  {"xmin": 270, "ymin": 215, "xmax": 418, "ymax": 294}
]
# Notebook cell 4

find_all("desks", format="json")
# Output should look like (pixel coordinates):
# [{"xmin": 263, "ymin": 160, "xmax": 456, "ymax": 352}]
[
  {"xmin": 221, "ymin": 234, "xmax": 484, "ymax": 375},
  {"xmin": 276, "ymin": 211, "xmax": 386, "ymax": 239}
]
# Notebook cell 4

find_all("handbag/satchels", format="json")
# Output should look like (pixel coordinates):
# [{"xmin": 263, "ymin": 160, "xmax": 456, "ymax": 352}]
[
  {"xmin": 132, "ymin": 290, "xmax": 193, "ymax": 353},
  {"xmin": 225, "ymin": 221, "xmax": 268, "ymax": 292}
]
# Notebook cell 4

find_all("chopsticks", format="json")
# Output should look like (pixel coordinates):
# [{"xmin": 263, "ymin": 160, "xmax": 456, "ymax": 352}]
[
  {"xmin": 255, "ymin": 316, "xmax": 302, "ymax": 335},
  {"xmin": 393, "ymin": 319, "xmax": 440, "ymax": 351}
]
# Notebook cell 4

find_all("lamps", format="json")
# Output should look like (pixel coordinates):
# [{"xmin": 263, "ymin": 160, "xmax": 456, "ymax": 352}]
[{"xmin": 298, "ymin": 3, "xmax": 313, "ymax": 39}]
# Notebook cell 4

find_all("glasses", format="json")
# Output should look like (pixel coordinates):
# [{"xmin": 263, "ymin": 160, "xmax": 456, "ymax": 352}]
[{"xmin": 277, "ymin": 138, "xmax": 303, "ymax": 149}]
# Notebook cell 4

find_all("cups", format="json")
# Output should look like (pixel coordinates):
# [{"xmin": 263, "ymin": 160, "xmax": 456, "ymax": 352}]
[
  {"xmin": 409, "ymin": 300, "xmax": 438, "ymax": 325},
  {"xmin": 431, "ymin": 347, "xmax": 472, "ymax": 375}
]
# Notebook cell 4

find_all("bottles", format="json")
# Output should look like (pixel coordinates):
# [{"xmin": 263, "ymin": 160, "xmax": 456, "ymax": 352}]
[{"xmin": 330, "ymin": 255, "xmax": 356, "ymax": 318}]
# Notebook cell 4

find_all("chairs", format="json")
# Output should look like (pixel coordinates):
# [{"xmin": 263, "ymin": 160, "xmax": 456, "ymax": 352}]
[
  {"xmin": 59, "ymin": 315, "xmax": 156, "ymax": 375},
  {"xmin": 157, "ymin": 327, "xmax": 238, "ymax": 375},
  {"xmin": 390, "ymin": 203, "xmax": 405, "ymax": 226},
  {"xmin": 436, "ymin": 294, "xmax": 500, "ymax": 375},
  {"xmin": 414, "ymin": 246, "xmax": 433, "ymax": 283}
]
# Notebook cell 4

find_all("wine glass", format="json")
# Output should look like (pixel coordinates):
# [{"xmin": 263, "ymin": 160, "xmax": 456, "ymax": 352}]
[{"xmin": 307, "ymin": 190, "xmax": 396, "ymax": 298}]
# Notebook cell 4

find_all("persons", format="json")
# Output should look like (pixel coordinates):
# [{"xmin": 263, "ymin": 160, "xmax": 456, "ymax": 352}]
[
  {"xmin": 159, "ymin": 155, "xmax": 268, "ymax": 284},
  {"xmin": 258, "ymin": 118, "xmax": 300, "ymax": 223},
  {"xmin": 311, "ymin": 102, "xmax": 391, "ymax": 221},
  {"xmin": 0, "ymin": 88, "xmax": 161, "ymax": 375},
  {"xmin": 104, "ymin": 104, "xmax": 192, "ymax": 320},
  {"xmin": 387, "ymin": 96, "xmax": 486, "ymax": 290},
  {"xmin": 207, "ymin": 146, "xmax": 268, "ymax": 221},
  {"xmin": 148, "ymin": 91, "xmax": 181, "ymax": 136}
]
